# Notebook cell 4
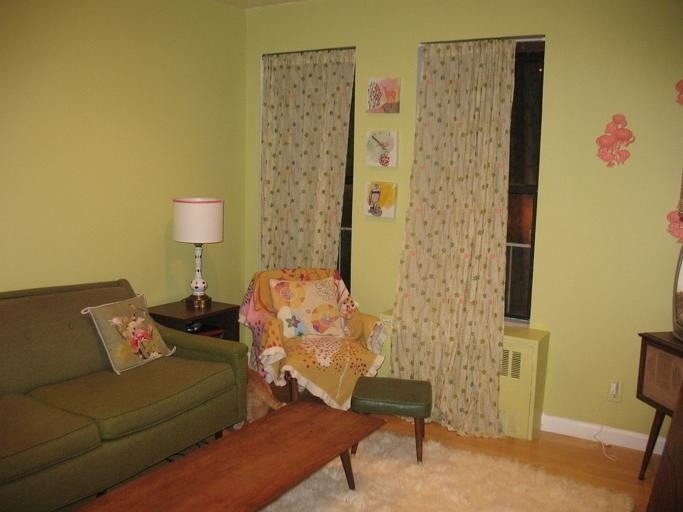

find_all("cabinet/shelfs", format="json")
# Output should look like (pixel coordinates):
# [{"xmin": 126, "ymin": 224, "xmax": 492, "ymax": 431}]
[{"xmin": 148, "ymin": 300, "xmax": 240, "ymax": 345}]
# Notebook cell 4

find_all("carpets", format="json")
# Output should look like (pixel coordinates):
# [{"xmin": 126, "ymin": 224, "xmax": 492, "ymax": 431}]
[{"xmin": 258, "ymin": 430, "xmax": 632, "ymax": 511}]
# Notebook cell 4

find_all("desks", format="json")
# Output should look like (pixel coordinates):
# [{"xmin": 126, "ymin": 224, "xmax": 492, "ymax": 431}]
[{"xmin": 636, "ymin": 332, "xmax": 683, "ymax": 480}]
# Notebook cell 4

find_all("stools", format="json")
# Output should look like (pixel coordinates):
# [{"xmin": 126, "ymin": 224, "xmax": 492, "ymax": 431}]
[{"xmin": 350, "ymin": 377, "xmax": 432, "ymax": 466}]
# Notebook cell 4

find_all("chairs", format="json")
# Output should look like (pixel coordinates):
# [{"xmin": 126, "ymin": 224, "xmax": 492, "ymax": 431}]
[{"xmin": 238, "ymin": 268, "xmax": 387, "ymax": 401}]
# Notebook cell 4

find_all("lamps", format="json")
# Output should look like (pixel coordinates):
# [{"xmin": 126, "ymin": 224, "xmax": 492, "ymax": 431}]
[{"xmin": 173, "ymin": 198, "xmax": 223, "ymax": 308}]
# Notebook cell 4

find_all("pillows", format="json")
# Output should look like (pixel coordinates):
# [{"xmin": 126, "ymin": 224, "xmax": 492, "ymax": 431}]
[
  {"xmin": 272, "ymin": 277, "xmax": 347, "ymax": 338},
  {"xmin": 82, "ymin": 294, "xmax": 174, "ymax": 376}
]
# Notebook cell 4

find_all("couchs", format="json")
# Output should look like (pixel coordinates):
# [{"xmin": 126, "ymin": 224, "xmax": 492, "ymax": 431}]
[{"xmin": 0, "ymin": 280, "xmax": 248, "ymax": 512}]
